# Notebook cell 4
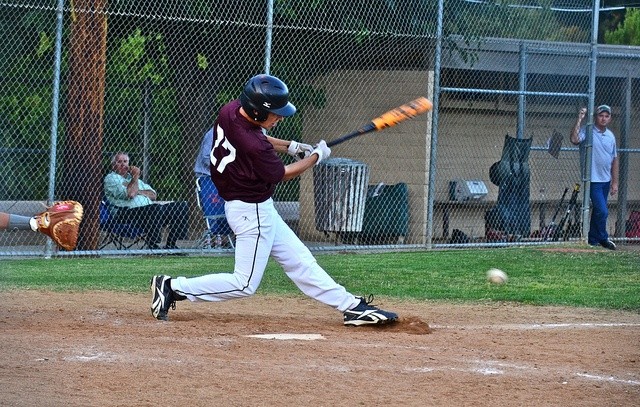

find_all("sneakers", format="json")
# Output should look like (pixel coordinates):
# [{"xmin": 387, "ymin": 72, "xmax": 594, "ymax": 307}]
[
  {"xmin": 147, "ymin": 245, "xmax": 164, "ymax": 255},
  {"xmin": 166, "ymin": 245, "xmax": 188, "ymax": 255},
  {"xmin": 600, "ymin": 238, "xmax": 616, "ymax": 250},
  {"xmin": 343, "ymin": 294, "xmax": 398, "ymax": 325},
  {"xmin": 151, "ymin": 275, "xmax": 187, "ymax": 320}
]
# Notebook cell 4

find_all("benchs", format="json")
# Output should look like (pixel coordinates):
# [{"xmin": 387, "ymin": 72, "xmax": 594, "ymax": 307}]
[{"xmin": 431, "ymin": 198, "xmax": 639, "ymax": 242}]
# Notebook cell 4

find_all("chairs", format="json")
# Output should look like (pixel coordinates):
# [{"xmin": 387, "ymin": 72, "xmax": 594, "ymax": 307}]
[
  {"xmin": 99, "ymin": 197, "xmax": 154, "ymax": 250},
  {"xmin": 192, "ymin": 174, "xmax": 235, "ymax": 249}
]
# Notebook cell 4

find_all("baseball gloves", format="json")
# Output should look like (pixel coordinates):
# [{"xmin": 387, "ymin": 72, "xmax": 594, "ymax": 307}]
[{"xmin": 33, "ymin": 200, "xmax": 84, "ymax": 252}]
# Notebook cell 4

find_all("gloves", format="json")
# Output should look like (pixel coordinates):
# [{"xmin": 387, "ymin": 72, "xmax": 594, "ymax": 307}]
[
  {"xmin": 309, "ymin": 139, "xmax": 331, "ymax": 166},
  {"xmin": 287, "ymin": 140, "xmax": 314, "ymax": 161}
]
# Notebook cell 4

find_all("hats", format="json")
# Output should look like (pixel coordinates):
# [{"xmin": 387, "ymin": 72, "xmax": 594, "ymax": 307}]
[{"xmin": 596, "ymin": 104, "xmax": 612, "ymax": 113}]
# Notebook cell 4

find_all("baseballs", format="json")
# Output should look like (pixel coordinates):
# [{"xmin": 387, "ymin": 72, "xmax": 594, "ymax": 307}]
[{"xmin": 486, "ymin": 268, "xmax": 508, "ymax": 284}]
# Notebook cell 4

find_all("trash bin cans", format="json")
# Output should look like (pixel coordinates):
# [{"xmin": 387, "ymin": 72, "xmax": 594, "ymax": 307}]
[{"xmin": 311, "ymin": 157, "xmax": 370, "ymax": 234}]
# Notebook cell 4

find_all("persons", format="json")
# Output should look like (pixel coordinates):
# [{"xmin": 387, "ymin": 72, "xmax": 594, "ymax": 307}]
[
  {"xmin": 570, "ymin": 102, "xmax": 620, "ymax": 251},
  {"xmin": 102, "ymin": 152, "xmax": 190, "ymax": 255},
  {"xmin": 0, "ymin": 212, "xmax": 38, "ymax": 232},
  {"xmin": 195, "ymin": 121, "xmax": 227, "ymax": 248},
  {"xmin": 150, "ymin": 73, "xmax": 399, "ymax": 325}
]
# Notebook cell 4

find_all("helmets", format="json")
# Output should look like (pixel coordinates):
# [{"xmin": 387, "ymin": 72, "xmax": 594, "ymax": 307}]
[{"xmin": 241, "ymin": 73, "xmax": 297, "ymax": 122}]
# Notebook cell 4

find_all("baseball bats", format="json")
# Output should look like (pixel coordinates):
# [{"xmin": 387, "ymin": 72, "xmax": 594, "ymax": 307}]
[
  {"xmin": 298, "ymin": 96, "xmax": 433, "ymax": 159},
  {"xmin": 540, "ymin": 187, "xmax": 569, "ymax": 242},
  {"xmin": 551, "ymin": 183, "xmax": 581, "ymax": 242}
]
